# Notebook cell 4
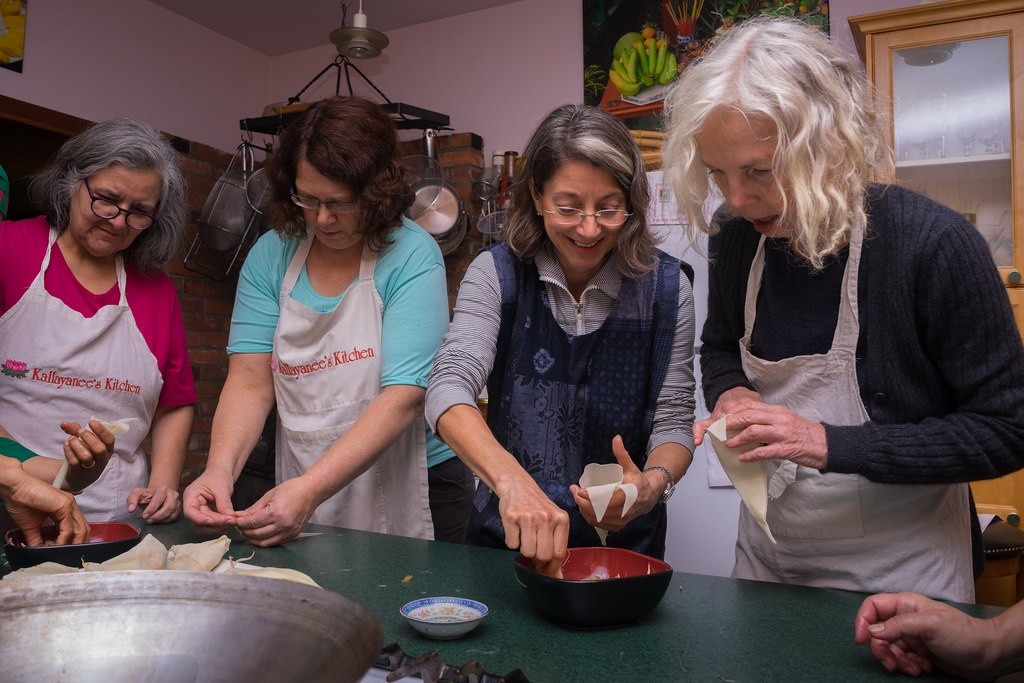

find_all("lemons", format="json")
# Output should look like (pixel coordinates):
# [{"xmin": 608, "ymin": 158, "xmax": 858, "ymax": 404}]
[{"xmin": 629, "ymin": 27, "xmax": 670, "ymax": 51}]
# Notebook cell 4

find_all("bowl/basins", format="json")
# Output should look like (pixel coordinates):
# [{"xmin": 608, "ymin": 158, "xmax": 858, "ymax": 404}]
[
  {"xmin": 5, "ymin": 521, "xmax": 143, "ymax": 570},
  {"xmin": 399, "ymin": 596, "xmax": 489, "ymax": 640},
  {"xmin": 512, "ymin": 546, "xmax": 674, "ymax": 623}
]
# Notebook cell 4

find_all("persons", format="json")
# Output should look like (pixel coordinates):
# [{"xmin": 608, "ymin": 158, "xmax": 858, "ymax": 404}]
[
  {"xmin": 853, "ymin": 592, "xmax": 1024, "ymax": 675},
  {"xmin": 0, "ymin": 121, "xmax": 198, "ymax": 524},
  {"xmin": 659, "ymin": 14, "xmax": 1024, "ymax": 603},
  {"xmin": 0, "ymin": 421, "xmax": 115, "ymax": 550},
  {"xmin": 424, "ymin": 102, "xmax": 695, "ymax": 564},
  {"xmin": 182, "ymin": 95, "xmax": 474, "ymax": 550}
]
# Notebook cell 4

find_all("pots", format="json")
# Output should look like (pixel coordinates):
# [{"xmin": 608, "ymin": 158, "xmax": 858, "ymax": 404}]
[
  {"xmin": 196, "ymin": 167, "xmax": 259, "ymax": 252},
  {"xmin": 2, "ymin": 570, "xmax": 384, "ymax": 683},
  {"xmin": 404, "ymin": 128, "xmax": 467, "ymax": 256}
]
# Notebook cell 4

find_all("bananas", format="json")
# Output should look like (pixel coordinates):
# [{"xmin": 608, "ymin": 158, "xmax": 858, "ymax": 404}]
[{"xmin": 607, "ymin": 39, "xmax": 678, "ymax": 97}]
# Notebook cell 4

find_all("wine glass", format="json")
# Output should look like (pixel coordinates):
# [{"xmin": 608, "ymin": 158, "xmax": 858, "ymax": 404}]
[{"xmin": 894, "ymin": 93, "xmax": 1010, "ymax": 160}]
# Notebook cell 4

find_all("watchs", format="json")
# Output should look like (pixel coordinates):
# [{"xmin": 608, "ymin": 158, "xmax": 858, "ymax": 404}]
[{"xmin": 642, "ymin": 466, "xmax": 676, "ymax": 504}]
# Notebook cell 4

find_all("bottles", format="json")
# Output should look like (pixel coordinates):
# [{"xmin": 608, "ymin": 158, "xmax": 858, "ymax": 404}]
[{"xmin": 482, "ymin": 148, "xmax": 521, "ymax": 249}]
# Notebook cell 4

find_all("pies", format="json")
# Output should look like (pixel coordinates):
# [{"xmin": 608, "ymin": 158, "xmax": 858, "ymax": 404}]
[{"xmin": 0, "ymin": 532, "xmax": 320, "ymax": 589}]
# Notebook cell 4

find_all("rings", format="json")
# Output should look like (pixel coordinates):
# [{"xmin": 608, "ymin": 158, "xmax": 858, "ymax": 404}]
[{"xmin": 82, "ymin": 461, "xmax": 96, "ymax": 469}]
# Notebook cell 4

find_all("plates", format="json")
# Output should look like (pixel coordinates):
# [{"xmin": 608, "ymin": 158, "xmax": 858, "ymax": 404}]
[{"xmin": 621, "ymin": 75, "xmax": 680, "ymax": 106}]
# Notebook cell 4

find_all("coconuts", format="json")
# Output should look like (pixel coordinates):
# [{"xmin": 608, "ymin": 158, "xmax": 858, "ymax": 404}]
[{"xmin": 613, "ymin": 32, "xmax": 643, "ymax": 58}]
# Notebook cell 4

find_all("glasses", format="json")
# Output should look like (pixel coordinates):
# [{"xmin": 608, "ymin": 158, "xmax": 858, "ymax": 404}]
[
  {"xmin": 534, "ymin": 187, "xmax": 636, "ymax": 226},
  {"xmin": 290, "ymin": 188, "xmax": 359, "ymax": 213},
  {"xmin": 83, "ymin": 177, "xmax": 160, "ymax": 231}
]
